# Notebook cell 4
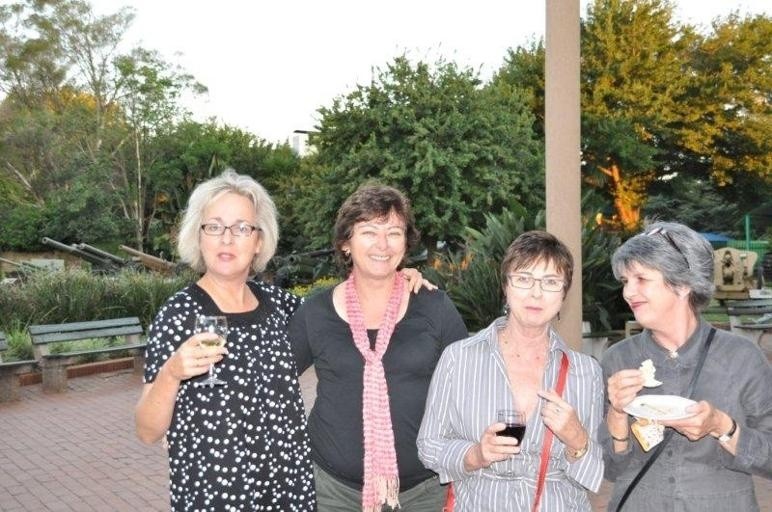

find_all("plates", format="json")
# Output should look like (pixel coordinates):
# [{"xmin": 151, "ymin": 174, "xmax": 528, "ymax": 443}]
[{"xmin": 621, "ymin": 393, "xmax": 702, "ymax": 422}]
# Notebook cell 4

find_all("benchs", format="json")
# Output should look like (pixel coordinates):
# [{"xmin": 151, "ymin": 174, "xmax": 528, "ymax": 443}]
[
  {"xmin": 698, "ymin": 300, "xmax": 772, "ymax": 345},
  {"xmin": 28, "ymin": 315, "xmax": 150, "ymax": 391}
]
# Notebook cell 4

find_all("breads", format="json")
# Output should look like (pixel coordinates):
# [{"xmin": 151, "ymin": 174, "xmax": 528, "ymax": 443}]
[{"xmin": 639, "ymin": 359, "xmax": 663, "ymax": 387}]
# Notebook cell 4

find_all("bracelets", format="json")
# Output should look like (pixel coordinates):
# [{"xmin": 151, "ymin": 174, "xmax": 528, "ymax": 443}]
[
  {"xmin": 567, "ymin": 439, "xmax": 588, "ymax": 459},
  {"xmin": 609, "ymin": 433, "xmax": 628, "ymax": 442}
]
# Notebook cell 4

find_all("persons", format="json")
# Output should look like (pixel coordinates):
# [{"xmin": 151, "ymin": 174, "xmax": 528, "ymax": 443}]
[
  {"xmin": 133, "ymin": 167, "xmax": 440, "ymax": 512},
  {"xmin": 598, "ymin": 220, "xmax": 772, "ymax": 512},
  {"xmin": 287, "ymin": 186, "xmax": 470, "ymax": 512},
  {"xmin": 416, "ymin": 231, "xmax": 605, "ymax": 512}
]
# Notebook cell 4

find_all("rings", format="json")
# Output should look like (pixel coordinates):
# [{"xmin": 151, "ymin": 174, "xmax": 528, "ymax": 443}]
[
  {"xmin": 196, "ymin": 358, "xmax": 200, "ymax": 367},
  {"xmin": 554, "ymin": 406, "xmax": 561, "ymax": 413}
]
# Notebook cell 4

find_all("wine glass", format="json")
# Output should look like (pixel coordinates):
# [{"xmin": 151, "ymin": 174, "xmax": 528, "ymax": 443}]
[
  {"xmin": 493, "ymin": 409, "xmax": 527, "ymax": 480},
  {"xmin": 192, "ymin": 314, "xmax": 229, "ymax": 388}
]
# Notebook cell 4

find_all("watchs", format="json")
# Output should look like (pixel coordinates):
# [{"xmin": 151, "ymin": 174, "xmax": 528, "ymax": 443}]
[{"xmin": 714, "ymin": 417, "xmax": 737, "ymax": 443}]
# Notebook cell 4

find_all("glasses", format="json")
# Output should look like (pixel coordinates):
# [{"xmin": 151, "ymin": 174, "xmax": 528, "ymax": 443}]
[
  {"xmin": 640, "ymin": 227, "xmax": 690, "ymax": 269},
  {"xmin": 200, "ymin": 224, "xmax": 260, "ymax": 236},
  {"xmin": 505, "ymin": 274, "xmax": 567, "ymax": 291}
]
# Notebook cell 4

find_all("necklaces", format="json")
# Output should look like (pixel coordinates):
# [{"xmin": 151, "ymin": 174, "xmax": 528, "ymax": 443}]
[
  {"xmin": 649, "ymin": 322, "xmax": 699, "ymax": 360},
  {"xmin": 500, "ymin": 330, "xmax": 546, "ymax": 362}
]
